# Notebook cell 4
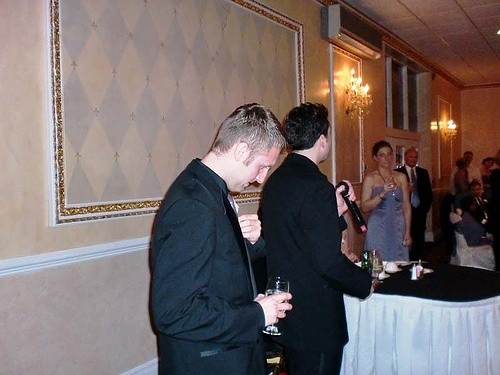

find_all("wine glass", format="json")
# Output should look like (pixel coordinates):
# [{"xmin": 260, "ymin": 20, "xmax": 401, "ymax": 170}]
[
  {"xmin": 388, "ymin": 178, "xmax": 398, "ymax": 196},
  {"xmin": 262, "ymin": 275, "xmax": 290, "ymax": 337}
]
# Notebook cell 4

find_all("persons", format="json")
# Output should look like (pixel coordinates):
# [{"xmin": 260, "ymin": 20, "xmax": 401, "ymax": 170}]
[
  {"xmin": 147, "ymin": 104, "xmax": 292, "ymax": 375},
  {"xmin": 395, "ymin": 146, "xmax": 434, "ymax": 263},
  {"xmin": 450, "ymin": 151, "xmax": 500, "ymax": 273},
  {"xmin": 361, "ymin": 140, "xmax": 411, "ymax": 264},
  {"xmin": 261, "ymin": 102, "xmax": 378, "ymax": 375}
]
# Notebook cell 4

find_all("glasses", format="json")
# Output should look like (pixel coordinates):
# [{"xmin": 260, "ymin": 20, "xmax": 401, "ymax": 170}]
[{"xmin": 375, "ymin": 152, "xmax": 393, "ymax": 158}]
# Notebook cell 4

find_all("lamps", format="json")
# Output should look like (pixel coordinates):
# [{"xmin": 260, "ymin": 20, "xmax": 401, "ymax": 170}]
[
  {"xmin": 442, "ymin": 120, "xmax": 458, "ymax": 141},
  {"xmin": 345, "ymin": 68, "xmax": 374, "ymax": 122}
]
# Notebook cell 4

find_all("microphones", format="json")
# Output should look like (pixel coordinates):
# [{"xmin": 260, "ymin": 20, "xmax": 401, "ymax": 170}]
[{"xmin": 336, "ymin": 182, "xmax": 368, "ymax": 232}]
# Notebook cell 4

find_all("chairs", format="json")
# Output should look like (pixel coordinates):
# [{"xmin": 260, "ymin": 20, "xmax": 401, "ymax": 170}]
[{"xmin": 448, "ymin": 212, "xmax": 496, "ymax": 271}]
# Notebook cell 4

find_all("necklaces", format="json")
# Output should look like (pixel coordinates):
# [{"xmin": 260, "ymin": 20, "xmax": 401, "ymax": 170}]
[{"xmin": 378, "ymin": 169, "xmax": 392, "ymax": 184}]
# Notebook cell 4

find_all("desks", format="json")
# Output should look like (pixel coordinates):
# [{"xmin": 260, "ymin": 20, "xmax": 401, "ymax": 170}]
[{"xmin": 341, "ymin": 260, "xmax": 500, "ymax": 375}]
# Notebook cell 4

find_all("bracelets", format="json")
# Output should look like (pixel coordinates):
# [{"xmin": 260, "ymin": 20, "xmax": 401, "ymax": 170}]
[{"xmin": 379, "ymin": 192, "xmax": 386, "ymax": 201}]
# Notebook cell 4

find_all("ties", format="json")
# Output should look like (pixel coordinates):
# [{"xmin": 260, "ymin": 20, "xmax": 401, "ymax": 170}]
[
  {"xmin": 477, "ymin": 196, "xmax": 488, "ymax": 215},
  {"xmin": 226, "ymin": 193, "xmax": 257, "ymax": 299},
  {"xmin": 411, "ymin": 167, "xmax": 419, "ymax": 207}
]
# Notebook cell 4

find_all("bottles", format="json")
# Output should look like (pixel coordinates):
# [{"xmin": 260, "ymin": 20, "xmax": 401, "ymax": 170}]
[
  {"xmin": 411, "ymin": 259, "xmax": 423, "ymax": 280},
  {"xmin": 370, "ymin": 249, "xmax": 383, "ymax": 282}
]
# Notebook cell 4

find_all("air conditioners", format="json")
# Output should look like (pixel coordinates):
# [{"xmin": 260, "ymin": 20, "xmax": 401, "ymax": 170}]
[{"xmin": 328, "ymin": 3, "xmax": 382, "ymax": 62}]
